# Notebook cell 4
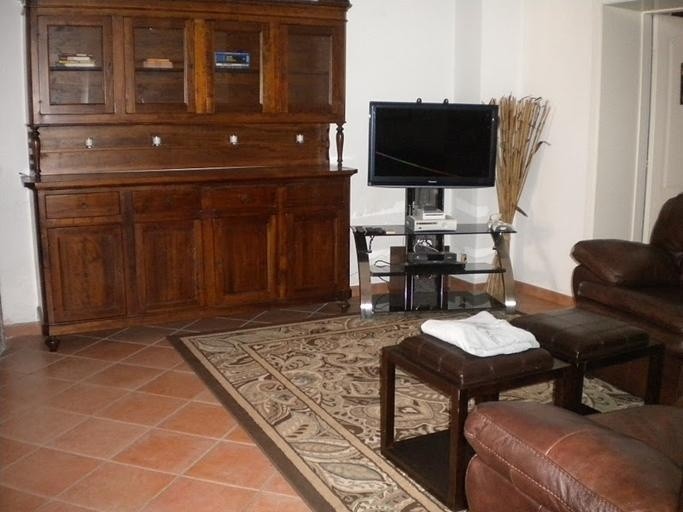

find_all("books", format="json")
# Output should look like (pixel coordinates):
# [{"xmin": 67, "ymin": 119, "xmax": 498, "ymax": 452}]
[
  {"xmin": 215, "ymin": 53, "xmax": 249, "ymax": 67},
  {"xmin": 55, "ymin": 52, "xmax": 96, "ymax": 68},
  {"xmin": 145, "ymin": 57, "xmax": 170, "ymax": 62},
  {"xmin": 141, "ymin": 60, "xmax": 173, "ymax": 68}
]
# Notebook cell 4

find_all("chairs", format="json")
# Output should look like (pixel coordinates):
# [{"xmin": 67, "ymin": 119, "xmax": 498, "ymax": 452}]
[
  {"xmin": 571, "ymin": 193, "xmax": 683, "ymax": 404},
  {"xmin": 462, "ymin": 397, "xmax": 683, "ymax": 512}
]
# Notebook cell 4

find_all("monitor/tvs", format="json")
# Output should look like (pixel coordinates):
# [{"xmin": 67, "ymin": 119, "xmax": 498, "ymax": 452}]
[{"xmin": 366, "ymin": 102, "xmax": 499, "ymax": 188}]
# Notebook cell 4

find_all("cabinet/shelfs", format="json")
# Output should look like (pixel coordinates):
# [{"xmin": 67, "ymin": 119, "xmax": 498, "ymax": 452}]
[{"xmin": 20, "ymin": 0, "xmax": 358, "ymax": 352}]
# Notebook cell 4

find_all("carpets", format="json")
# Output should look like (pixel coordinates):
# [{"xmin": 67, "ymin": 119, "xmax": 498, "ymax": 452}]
[{"xmin": 167, "ymin": 307, "xmax": 646, "ymax": 512}]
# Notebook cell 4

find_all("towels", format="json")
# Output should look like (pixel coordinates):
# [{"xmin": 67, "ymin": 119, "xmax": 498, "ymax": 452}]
[{"xmin": 420, "ymin": 310, "xmax": 541, "ymax": 357}]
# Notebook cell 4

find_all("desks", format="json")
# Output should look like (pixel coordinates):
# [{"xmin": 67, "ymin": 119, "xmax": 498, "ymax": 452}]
[{"xmin": 351, "ymin": 223, "xmax": 516, "ymax": 319}]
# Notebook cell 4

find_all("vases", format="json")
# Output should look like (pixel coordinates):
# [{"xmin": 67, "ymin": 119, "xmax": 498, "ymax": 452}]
[{"xmin": 488, "ymin": 213, "xmax": 517, "ymax": 307}]
